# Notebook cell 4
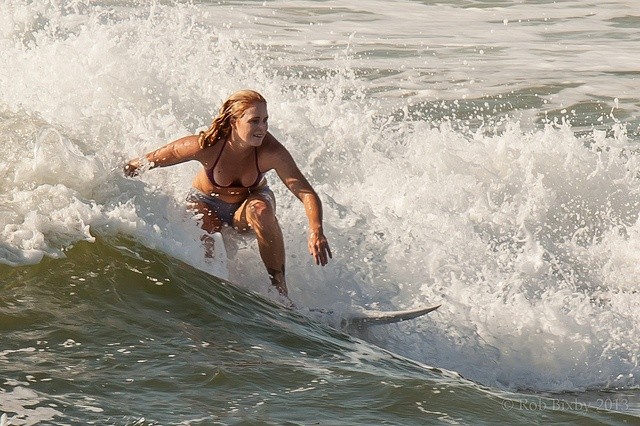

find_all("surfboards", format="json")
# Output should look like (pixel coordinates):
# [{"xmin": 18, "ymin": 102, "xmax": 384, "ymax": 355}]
[{"xmin": 301, "ymin": 304, "xmax": 444, "ymax": 327}]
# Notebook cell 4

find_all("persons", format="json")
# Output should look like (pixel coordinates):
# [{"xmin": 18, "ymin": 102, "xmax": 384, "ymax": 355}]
[{"xmin": 123, "ymin": 89, "xmax": 332, "ymax": 309}]
